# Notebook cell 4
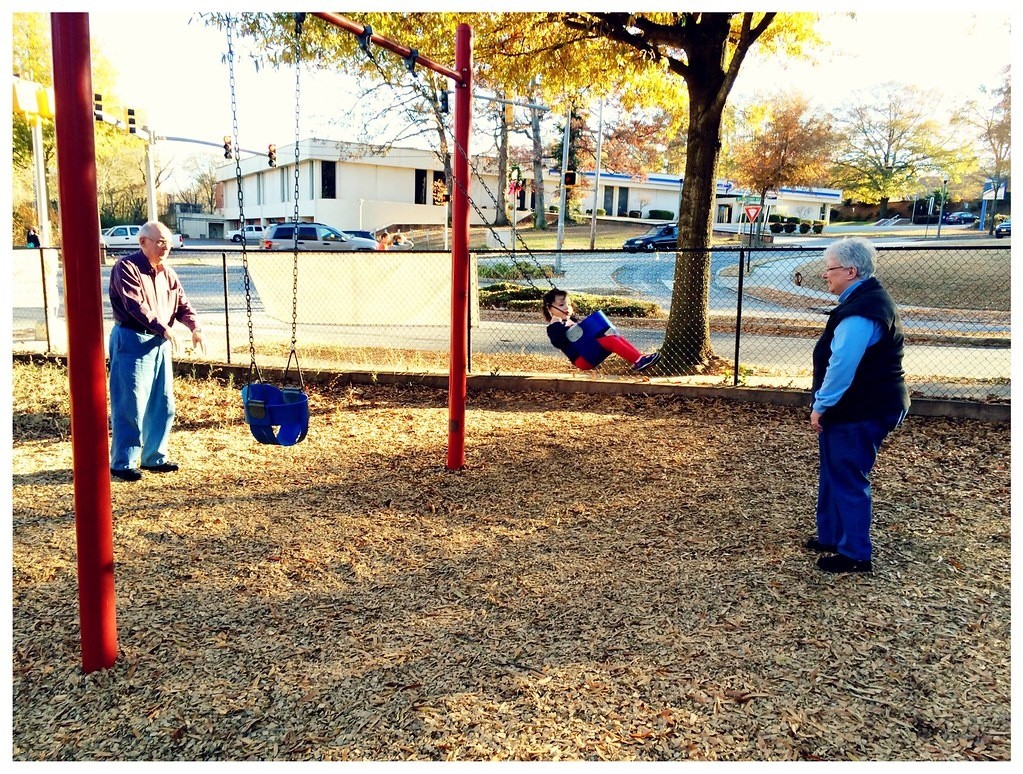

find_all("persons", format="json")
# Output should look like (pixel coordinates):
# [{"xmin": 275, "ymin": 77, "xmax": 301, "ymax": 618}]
[
  {"xmin": 377, "ymin": 233, "xmax": 415, "ymax": 251},
  {"xmin": 108, "ymin": 221, "xmax": 207, "ymax": 483},
  {"xmin": 801, "ymin": 237, "xmax": 913, "ymax": 573},
  {"xmin": 26, "ymin": 227, "xmax": 42, "ymax": 248},
  {"xmin": 542, "ymin": 289, "xmax": 661, "ymax": 372}
]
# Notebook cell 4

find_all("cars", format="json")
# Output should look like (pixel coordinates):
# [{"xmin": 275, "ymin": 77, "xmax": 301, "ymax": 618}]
[
  {"xmin": 101, "ymin": 228, "xmax": 127, "ymax": 235},
  {"xmin": 995, "ymin": 215, "xmax": 1011, "ymax": 239},
  {"xmin": 946, "ymin": 212, "xmax": 980, "ymax": 225},
  {"xmin": 100, "ymin": 225, "xmax": 185, "ymax": 252}
]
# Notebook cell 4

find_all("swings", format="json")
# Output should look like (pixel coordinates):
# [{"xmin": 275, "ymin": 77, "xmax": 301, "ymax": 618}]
[
  {"xmin": 360, "ymin": 42, "xmax": 619, "ymax": 367},
  {"xmin": 225, "ymin": 12, "xmax": 310, "ymax": 446}
]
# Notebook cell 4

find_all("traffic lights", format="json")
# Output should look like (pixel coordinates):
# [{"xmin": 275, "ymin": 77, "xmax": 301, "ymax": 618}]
[
  {"xmin": 125, "ymin": 108, "xmax": 135, "ymax": 134},
  {"xmin": 268, "ymin": 143, "xmax": 277, "ymax": 167},
  {"xmin": 439, "ymin": 92, "xmax": 448, "ymax": 113},
  {"xmin": 93, "ymin": 92, "xmax": 103, "ymax": 122},
  {"xmin": 224, "ymin": 136, "xmax": 232, "ymax": 158},
  {"xmin": 564, "ymin": 172, "xmax": 576, "ymax": 186}
]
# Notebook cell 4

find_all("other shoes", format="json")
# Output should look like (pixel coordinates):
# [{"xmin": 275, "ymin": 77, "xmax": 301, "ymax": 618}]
[
  {"xmin": 140, "ymin": 463, "xmax": 179, "ymax": 473},
  {"xmin": 806, "ymin": 537, "xmax": 837, "ymax": 553},
  {"xmin": 110, "ymin": 468, "xmax": 140, "ymax": 481},
  {"xmin": 634, "ymin": 353, "xmax": 662, "ymax": 371},
  {"xmin": 817, "ymin": 554, "xmax": 871, "ymax": 573}
]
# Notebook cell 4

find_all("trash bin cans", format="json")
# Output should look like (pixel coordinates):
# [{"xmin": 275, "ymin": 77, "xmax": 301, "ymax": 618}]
[{"xmin": 200, "ymin": 234, "xmax": 205, "ymax": 239}]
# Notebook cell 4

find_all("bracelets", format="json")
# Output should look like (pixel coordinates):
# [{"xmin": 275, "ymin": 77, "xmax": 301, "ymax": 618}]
[{"xmin": 194, "ymin": 327, "xmax": 202, "ymax": 333}]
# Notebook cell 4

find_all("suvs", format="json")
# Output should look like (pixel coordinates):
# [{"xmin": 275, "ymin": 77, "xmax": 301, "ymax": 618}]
[
  {"xmin": 622, "ymin": 222, "xmax": 680, "ymax": 250},
  {"xmin": 260, "ymin": 222, "xmax": 277, "ymax": 249},
  {"xmin": 261, "ymin": 222, "xmax": 380, "ymax": 250},
  {"xmin": 226, "ymin": 224, "xmax": 266, "ymax": 242}
]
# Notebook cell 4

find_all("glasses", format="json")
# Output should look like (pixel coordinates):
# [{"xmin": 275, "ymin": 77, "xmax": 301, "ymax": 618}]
[
  {"xmin": 826, "ymin": 266, "xmax": 843, "ymax": 271},
  {"xmin": 145, "ymin": 237, "xmax": 175, "ymax": 248}
]
